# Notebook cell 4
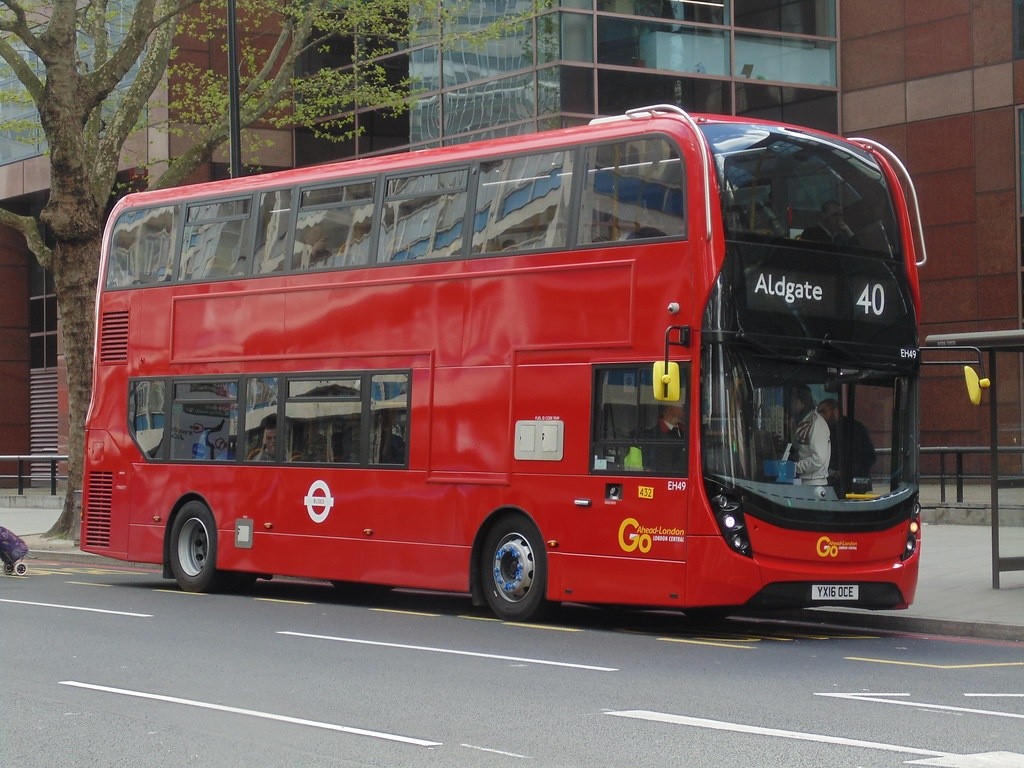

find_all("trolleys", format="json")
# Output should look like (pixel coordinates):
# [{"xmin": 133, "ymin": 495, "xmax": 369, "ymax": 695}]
[{"xmin": 0, "ymin": 544, "xmax": 37, "ymax": 576}]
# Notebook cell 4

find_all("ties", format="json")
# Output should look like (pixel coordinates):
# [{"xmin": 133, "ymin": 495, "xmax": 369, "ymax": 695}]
[{"xmin": 672, "ymin": 427, "xmax": 679, "ymax": 438}]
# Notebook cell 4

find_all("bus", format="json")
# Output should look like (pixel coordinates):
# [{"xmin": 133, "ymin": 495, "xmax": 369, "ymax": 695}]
[{"xmin": 79, "ymin": 103, "xmax": 990, "ymax": 622}]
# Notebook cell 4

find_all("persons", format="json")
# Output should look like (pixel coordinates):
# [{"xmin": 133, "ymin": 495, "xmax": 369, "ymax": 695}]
[
  {"xmin": 803, "ymin": 198, "xmax": 861, "ymax": 246},
  {"xmin": 252, "ymin": 419, "xmax": 287, "ymax": 462},
  {"xmin": 818, "ymin": 398, "xmax": 879, "ymax": 495},
  {"xmin": 641, "ymin": 394, "xmax": 690, "ymax": 475},
  {"xmin": 787, "ymin": 381, "xmax": 832, "ymax": 487}
]
